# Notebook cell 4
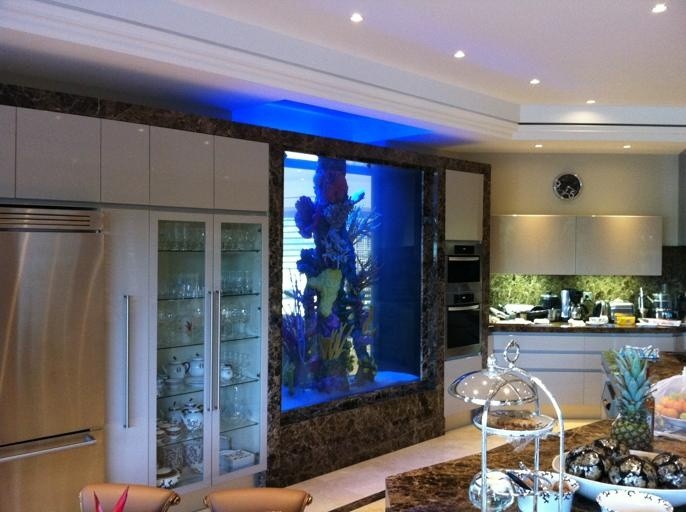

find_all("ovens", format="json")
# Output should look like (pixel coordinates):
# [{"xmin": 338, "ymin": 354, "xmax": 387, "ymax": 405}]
[{"xmin": 441, "ymin": 241, "xmax": 484, "ymax": 362}]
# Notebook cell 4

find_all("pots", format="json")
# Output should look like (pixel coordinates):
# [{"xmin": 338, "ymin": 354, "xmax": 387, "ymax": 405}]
[{"xmin": 541, "ymin": 290, "xmax": 559, "ymax": 322}]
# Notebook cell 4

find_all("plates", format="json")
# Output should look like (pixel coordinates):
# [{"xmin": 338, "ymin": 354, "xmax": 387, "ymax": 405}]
[{"xmin": 651, "ymin": 411, "xmax": 686, "ymax": 429}]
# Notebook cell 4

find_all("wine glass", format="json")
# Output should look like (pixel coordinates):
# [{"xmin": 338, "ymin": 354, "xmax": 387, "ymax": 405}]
[
  {"xmin": 224, "ymin": 351, "xmax": 249, "ymax": 382},
  {"xmin": 160, "ymin": 222, "xmax": 256, "ymax": 341}
]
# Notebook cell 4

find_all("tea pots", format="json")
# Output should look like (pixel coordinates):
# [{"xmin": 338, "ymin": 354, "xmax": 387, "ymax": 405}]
[
  {"xmin": 159, "ymin": 398, "xmax": 205, "ymax": 430},
  {"xmin": 162, "ymin": 351, "xmax": 233, "ymax": 381}
]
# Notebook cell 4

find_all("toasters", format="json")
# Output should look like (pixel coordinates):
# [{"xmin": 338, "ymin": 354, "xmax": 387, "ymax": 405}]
[{"xmin": 609, "ymin": 304, "xmax": 635, "ymax": 322}]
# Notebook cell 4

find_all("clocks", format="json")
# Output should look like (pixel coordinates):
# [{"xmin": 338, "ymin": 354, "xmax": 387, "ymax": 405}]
[{"xmin": 554, "ymin": 172, "xmax": 585, "ymax": 202}]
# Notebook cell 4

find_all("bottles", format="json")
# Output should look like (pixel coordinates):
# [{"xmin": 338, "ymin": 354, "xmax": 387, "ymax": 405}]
[{"xmin": 221, "ymin": 387, "xmax": 250, "ymax": 427}]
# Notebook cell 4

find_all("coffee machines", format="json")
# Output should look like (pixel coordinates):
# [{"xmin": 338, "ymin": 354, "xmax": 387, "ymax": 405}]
[{"xmin": 568, "ymin": 288, "xmax": 588, "ymax": 321}]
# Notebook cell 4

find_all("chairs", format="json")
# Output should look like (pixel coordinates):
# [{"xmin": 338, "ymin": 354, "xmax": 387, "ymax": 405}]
[
  {"xmin": 77, "ymin": 484, "xmax": 182, "ymax": 511},
  {"xmin": 202, "ymin": 485, "xmax": 314, "ymax": 511}
]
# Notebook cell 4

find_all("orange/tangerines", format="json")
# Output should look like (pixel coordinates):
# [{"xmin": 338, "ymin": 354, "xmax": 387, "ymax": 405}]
[{"xmin": 654, "ymin": 394, "xmax": 685, "ymax": 418}]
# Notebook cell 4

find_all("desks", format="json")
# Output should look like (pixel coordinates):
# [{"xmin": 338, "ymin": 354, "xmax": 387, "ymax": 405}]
[{"xmin": 384, "ymin": 347, "xmax": 686, "ymax": 511}]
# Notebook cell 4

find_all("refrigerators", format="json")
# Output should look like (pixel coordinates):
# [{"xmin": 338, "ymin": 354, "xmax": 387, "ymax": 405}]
[{"xmin": 0, "ymin": 207, "xmax": 107, "ymax": 511}]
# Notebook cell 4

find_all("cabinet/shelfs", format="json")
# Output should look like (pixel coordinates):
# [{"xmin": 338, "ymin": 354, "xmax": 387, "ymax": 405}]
[
  {"xmin": 488, "ymin": 316, "xmax": 686, "ymax": 418},
  {"xmin": 0, "ymin": 106, "xmax": 269, "ymax": 212},
  {"xmin": 101, "ymin": 207, "xmax": 269, "ymax": 512}
]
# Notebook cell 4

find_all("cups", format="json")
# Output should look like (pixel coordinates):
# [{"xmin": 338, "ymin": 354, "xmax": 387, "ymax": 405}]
[{"xmin": 155, "ymin": 416, "xmax": 181, "ymax": 441}]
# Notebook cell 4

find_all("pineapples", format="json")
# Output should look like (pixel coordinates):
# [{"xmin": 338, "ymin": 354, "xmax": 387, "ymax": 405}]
[{"xmin": 610, "ymin": 347, "xmax": 653, "ymax": 450}]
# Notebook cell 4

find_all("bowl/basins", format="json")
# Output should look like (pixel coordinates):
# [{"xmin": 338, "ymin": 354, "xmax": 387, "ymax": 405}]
[
  {"xmin": 510, "ymin": 469, "xmax": 580, "ymax": 512},
  {"xmin": 551, "ymin": 446, "xmax": 685, "ymax": 507},
  {"xmin": 594, "ymin": 490, "xmax": 674, "ymax": 512}
]
